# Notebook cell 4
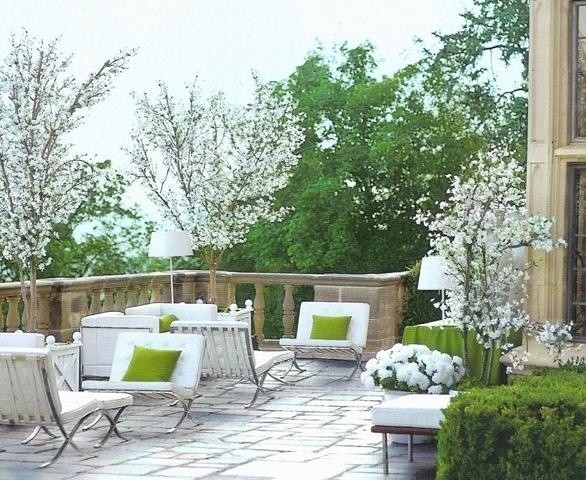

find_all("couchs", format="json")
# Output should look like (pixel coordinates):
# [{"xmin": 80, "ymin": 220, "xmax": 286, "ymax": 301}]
[{"xmin": 78, "ymin": 303, "xmax": 218, "ymax": 377}]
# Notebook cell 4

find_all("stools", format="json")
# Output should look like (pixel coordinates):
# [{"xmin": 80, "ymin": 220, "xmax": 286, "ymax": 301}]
[{"xmin": 370, "ymin": 393, "xmax": 455, "ymax": 475}]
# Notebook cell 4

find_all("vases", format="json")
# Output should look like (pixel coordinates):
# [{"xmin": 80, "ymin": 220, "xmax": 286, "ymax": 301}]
[{"xmin": 380, "ymin": 391, "xmax": 432, "ymax": 445}]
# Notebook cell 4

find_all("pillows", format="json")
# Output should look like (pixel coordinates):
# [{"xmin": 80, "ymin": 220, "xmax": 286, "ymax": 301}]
[
  {"xmin": 309, "ymin": 314, "xmax": 352, "ymax": 340},
  {"xmin": 121, "ymin": 344, "xmax": 181, "ymax": 382},
  {"xmin": 158, "ymin": 314, "xmax": 179, "ymax": 333}
]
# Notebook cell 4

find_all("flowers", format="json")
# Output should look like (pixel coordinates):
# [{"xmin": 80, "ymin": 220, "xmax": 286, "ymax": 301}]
[{"xmin": 360, "ymin": 342, "xmax": 466, "ymax": 395}]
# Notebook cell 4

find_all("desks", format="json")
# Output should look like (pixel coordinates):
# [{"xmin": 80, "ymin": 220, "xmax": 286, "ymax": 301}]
[{"xmin": 402, "ymin": 318, "xmax": 523, "ymax": 387}]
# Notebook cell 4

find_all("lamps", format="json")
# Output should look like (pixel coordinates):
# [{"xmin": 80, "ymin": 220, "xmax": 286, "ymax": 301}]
[
  {"xmin": 147, "ymin": 231, "xmax": 194, "ymax": 304},
  {"xmin": 417, "ymin": 256, "xmax": 452, "ymax": 320}
]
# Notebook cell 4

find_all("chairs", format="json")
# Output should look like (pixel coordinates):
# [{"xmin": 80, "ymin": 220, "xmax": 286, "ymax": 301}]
[
  {"xmin": 0, "ymin": 333, "xmax": 44, "ymax": 349},
  {"xmin": 170, "ymin": 319, "xmax": 294, "ymax": 409},
  {"xmin": 82, "ymin": 333, "xmax": 206, "ymax": 434},
  {"xmin": 0, "ymin": 348, "xmax": 98, "ymax": 468},
  {"xmin": 279, "ymin": 302, "xmax": 369, "ymax": 380}
]
